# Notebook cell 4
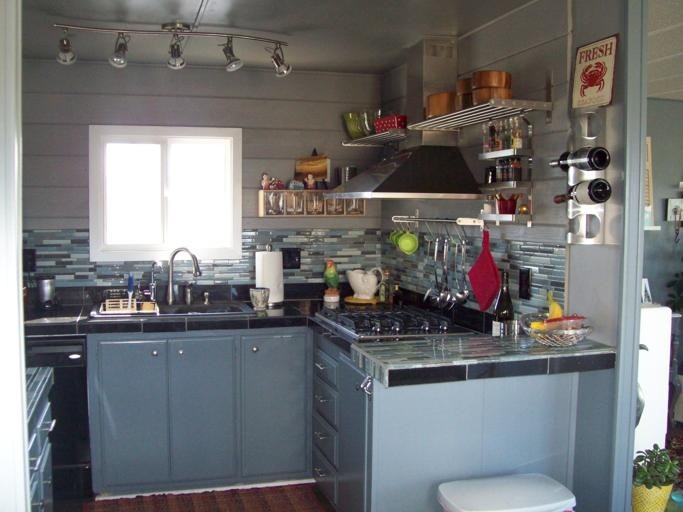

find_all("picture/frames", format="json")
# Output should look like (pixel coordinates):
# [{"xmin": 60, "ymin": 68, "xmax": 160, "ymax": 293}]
[{"xmin": 570, "ymin": 35, "xmax": 619, "ymax": 110}]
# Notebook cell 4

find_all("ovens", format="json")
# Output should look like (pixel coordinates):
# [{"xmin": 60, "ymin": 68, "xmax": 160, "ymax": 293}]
[{"xmin": 26, "ymin": 336, "xmax": 95, "ymax": 502}]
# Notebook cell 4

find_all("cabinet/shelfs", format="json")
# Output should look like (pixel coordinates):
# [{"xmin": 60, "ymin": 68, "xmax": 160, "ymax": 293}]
[
  {"xmin": 27, "ymin": 395, "xmax": 56, "ymax": 511},
  {"xmin": 95, "ymin": 335, "xmax": 235, "ymax": 487},
  {"xmin": 310, "ymin": 345, "xmax": 335, "ymax": 508},
  {"xmin": 337, "ymin": 360, "xmax": 366, "ymax": 512},
  {"xmin": 240, "ymin": 331, "xmax": 307, "ymax": 481},
  {"xmin": 256, "ymin": 187, "xmax": 367, "ymax": 218},
  {"xmin": 478, "ymin": 116, "xmax": 534, "ymax": 225}
]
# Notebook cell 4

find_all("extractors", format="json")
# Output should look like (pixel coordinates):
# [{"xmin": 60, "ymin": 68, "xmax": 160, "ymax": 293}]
[{"xmin": 331, "ymin": 32, "xmax": 487, "ymax": 203}]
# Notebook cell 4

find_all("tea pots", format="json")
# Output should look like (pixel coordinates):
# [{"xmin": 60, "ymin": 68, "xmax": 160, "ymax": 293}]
[{"xmin": 346, "ymin": 267, "xmax": 383, "ymax": 299}]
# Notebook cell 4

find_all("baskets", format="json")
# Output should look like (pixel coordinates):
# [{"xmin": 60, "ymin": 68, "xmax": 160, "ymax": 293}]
[
  {"xmin": 520, "ymin": 312, "xmax": 592, "ymax": 347},
  {"xmin": 106, "ymin": 288, "xmax": 136, "ymax": 310}
]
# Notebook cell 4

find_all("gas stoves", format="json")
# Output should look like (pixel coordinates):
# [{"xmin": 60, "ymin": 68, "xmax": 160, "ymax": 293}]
[{"xmin": 319, "ymin": 307, "xmax": 455, "ymax": 336}]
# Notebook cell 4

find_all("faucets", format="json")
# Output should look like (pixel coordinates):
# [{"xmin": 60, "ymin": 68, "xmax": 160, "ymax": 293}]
[
  {"xmin": 143, "ymin": 260, "xmax": 164, "ymax": 301},
  {"xmin": 167, "ymin": 248, "xmax": 203, "ymax": 305}
]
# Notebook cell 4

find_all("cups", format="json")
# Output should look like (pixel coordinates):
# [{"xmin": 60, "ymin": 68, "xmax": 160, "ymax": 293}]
[
  {"xmin": 494, "ymin": 199, "xmax": 518, "ymax": 214},
  {"xmin": 250, "ymin": 287, "xmax": 271, "ymax": 313},
  {"xmin": 38, "ymin": 275, "xmax": 56, "ymax": 304},
  {"xmin": 389, "ymin": 229, "xmax": 419, "ymax": 255},
  {"xmin": 266, "ymin": 191, "xmax": 364, "ymax": 215},
  {"xmin": 502, "ymin": 320, "xmax": 524, "ymax": 338}
]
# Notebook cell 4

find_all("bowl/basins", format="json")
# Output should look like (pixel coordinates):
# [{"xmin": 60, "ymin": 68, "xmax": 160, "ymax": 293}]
[
  {"xmin": 342, "ymin": 109, "xmax": 380, "ymax": 139},
  {"xmin": 425, "ymin": 69, "xmax": 512, "ymax": 119}
]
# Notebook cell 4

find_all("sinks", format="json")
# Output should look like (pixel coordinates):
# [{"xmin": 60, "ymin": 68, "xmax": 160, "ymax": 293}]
[
  {"xmin": 91, "ymin": 304, "xmax": 158, "ymax": 316},
  {"xmin": 156, "ymin": 304, "xmax": 253, "ymax": 315}
]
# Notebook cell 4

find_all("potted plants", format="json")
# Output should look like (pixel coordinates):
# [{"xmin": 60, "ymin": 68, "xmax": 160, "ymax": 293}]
[{"xmin": 631, "ymin": 444, "xmax": 681, "ymax": 510}]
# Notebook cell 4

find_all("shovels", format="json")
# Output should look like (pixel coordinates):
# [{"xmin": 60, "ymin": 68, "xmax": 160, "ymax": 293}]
[{"xmin": 423, "ymin": 237, "xmax": 439, "ymax": 303}]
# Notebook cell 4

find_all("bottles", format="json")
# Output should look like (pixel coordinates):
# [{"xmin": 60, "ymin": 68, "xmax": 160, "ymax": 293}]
[
  {"xmin": 547, "ymin": 147, "xmax": 613, "ymax": 170},
  {"xmin": 479, "ymin": 159, "xmax": 525, "ymax": 183},
  {"xmin": 380, "ymin": 268, "xmax": 401, "ymax": 308},
  {"xmin": 483, "ymin": 194, "xmax": 496, "ymax": 212},
  {"xmin": 480, "ymin": 117, "xmax": 531, "ymax": 152},
  {"xmin": 323, "ymin": 259, "xmax": 341, "ymax": 304},
  {"xmin": 552, "ymin": 180, "xmax": 615, "ymax": 206},
  {"xmin": 491, "ymin": 272, "xmax": 514, "ymax": 338}
]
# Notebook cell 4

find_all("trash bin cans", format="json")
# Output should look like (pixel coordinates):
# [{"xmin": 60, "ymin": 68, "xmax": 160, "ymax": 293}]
[{"xmin": 436, "ymin": 473, "xmax": 576, "ymax": 512}]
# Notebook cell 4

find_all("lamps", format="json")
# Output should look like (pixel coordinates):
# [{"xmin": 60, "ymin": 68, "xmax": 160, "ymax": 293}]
[{"xmin": 46, "ymin": 25, "xmax": 294, "ymax": 80}]
